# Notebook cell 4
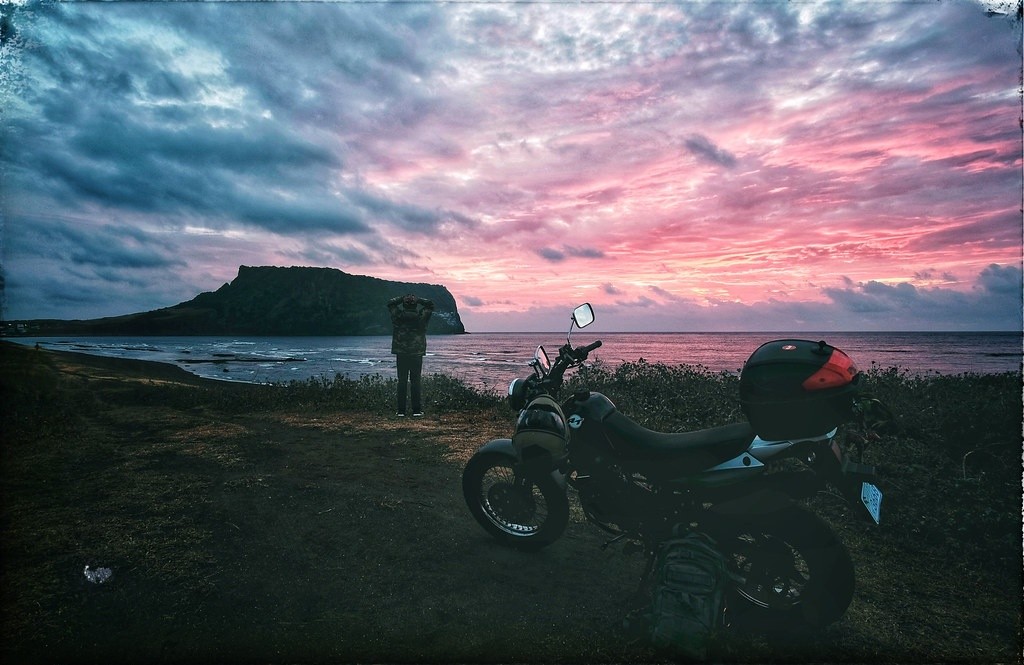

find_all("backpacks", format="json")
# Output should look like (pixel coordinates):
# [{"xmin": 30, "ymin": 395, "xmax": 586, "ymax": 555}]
[{"xmin": 620, "ymin": 521, "xmax": 732, "ymax": 665}]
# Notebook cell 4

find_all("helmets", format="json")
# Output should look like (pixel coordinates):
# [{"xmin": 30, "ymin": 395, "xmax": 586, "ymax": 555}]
[{"xmin": 511, "ymin": 394, "xmax": 571, "ymax": 474}]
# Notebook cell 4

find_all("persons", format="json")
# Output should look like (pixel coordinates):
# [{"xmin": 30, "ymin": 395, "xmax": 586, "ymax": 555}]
[{"xmin": 385, "ymin": 293, "xmax": 434, "ymax": 416}]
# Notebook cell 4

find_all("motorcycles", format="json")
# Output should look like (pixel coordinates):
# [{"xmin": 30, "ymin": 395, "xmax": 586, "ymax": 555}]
[{"xmin": 462, "ymin": 303, "xmax": 884, "ymax": 637}]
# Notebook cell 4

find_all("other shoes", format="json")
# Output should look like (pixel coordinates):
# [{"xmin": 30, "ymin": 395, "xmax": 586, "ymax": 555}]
[
  {"xmin": 413, "ymin": 411, "xmax": 425, "ymax": 417},
  {"xmin": 396, "ymin": 412, "xmax": 404, "ymax": 417}
]
